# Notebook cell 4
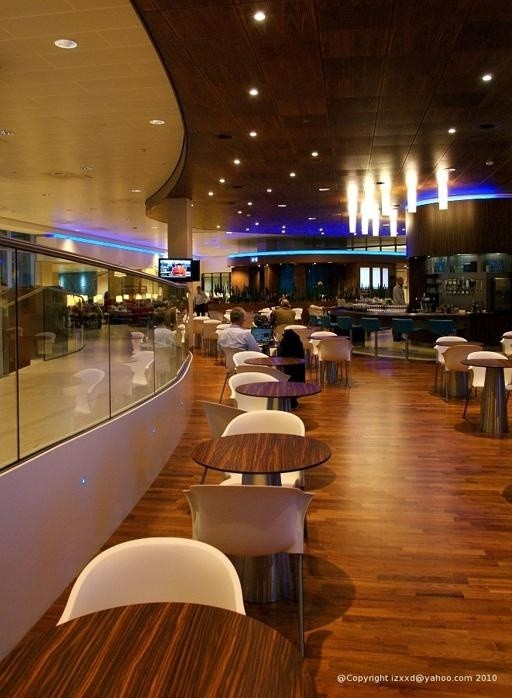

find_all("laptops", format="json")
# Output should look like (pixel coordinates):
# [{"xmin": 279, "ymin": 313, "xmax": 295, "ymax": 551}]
[{"xmin": 251, "ymin": 327, "xmax": 273, "ymax": 347}]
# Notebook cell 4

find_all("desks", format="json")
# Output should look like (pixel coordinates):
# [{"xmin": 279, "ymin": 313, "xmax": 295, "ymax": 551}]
[{"xmin": 21, "ymin": 335, "xmax": 52, "ymax": 360}]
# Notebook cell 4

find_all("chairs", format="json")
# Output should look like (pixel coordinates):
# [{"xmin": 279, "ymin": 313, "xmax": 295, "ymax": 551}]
[
  {"xmin": 228, "ymin": 372, "xmax": 279, "ymax": 412},
  {"xmin": 215, "ymin": 329, "xmax": 223, "ymax": 363},
  {"xmin": 261, "ymin": 310, "xmax": 272, "ymax": 321},
  {"xmin": 310, "ymin": 314, "xmax": 323, "ymax": 331},
  {"xmin": 285, "ymin": 325, "xmax": 307, "ymax": 349},
  {"xmin": 124, "ymin": 351, "xmax": 155, "ymax": 396},
  {"xmin": 308, "ymin": 305, "xmax": 322, "ymax": 316},
  {"xmin": 235, "ymin": 366, "xmax": 287, "ymax": 382},
  {"xmin": 503, "ymin": 331, "xmax": 512, "ymax": 339},
  {"xmin": 392, "ymin": 318, "xmax": 421, "ymax": 359},
  {"xmin": 216, "ymin": 324, "xmax": 231, "ymax": 329},
  {"xmin": 337, "ymin": 316, "xmax": 361, "ymax": 343},
  {"xmin": 224, "ymin": 309, "xmax": 232, "ymax": 314},
  {"xmin": 233, "ymin": 351, "xmax": 276, "ymax": 369},
  {"xmin": 269, "ymin": 347, "xmax": 278, "ymax": 357},
  {"xmin": 183, "ymin": 484, "xmax": 315, "ymax": 657},
  {"xmin": 321, "ymin": 315, "xmax": 337, "ymax": 332},
  {"xmin": 274, "ymin": 325, "xmax": 284, "ymax": 342},
  {"xmin": 223, "ymin": 314, "xmax": 231, "ymax": 324},
  {"xmin": 316, "ymin": 337, "xmax": 351, "ymax": 388},
  {"xmin": 309, "ymin": 332, "xmax": 338, "ymax": 378},
  {"xmin": 442, "ymin": 345, "xmax": 483, "ymax": 402},
  {"xmin": 435, "ymin": 336, "xmax": 469, "ymax": 392},
  {"xmin": 200, "ymin": 319, "xmax": 221, "ymax": 357},
  {"xmin": 362, "ymin": 318, "xmax": 391, "ymax": 359},
  {"xmin": 154, "ymin": 328, "xmax": 173, "ymax": 344},
  {"xmin": 258, "ymin": 308, "xmax": 270, "ymax": 313},
  {"xmin": 291, "ymin": 308, "xmax": 303, "ymax": 325},
  {"xmin": 501, "ymin": 338, "xmax": 512, "ymax": 355},
  {"xmin": 463, "ymin": 351, "xmax": 508, "ymax": 417},
  {"xmin": 192, "ymin": 317, "xmax": 211, "ymax": 349},
  {"xmin": 63, "ymin": 368, "xmax": 105, "ymax": 413},
  {"xmin": 7, "ymin": 326, "xmax": 24, "ymax": 339},
  {"xmin": 222, "ymin": 409, "xmax": 304, "ymax": 490},
  {"xmin": 129, "ymin": 331, "xmax": 145, "ymax": 354},
  {"xmin": 218, "ymin": 346, "xmax": 246, "ymax": 404},
  {"xmin": 58, "ymin": 537, "xmax": 248, "ymax": 624},
  {"xmin": 428, "ymin": 319, "xmax": 458, "ymax": 338},
  {"xmin": 199, "ymin": 400, "xmax": 246, "ymax": 484},
  {"xmin": 36, "ymin": 331, "xmax": 56, "ymax": 355}
]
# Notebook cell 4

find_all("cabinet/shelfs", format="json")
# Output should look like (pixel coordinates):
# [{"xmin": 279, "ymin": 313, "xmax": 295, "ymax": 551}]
[{"xmin": 423, "ymin": 257, "xmax": 512, "ymax": 345}]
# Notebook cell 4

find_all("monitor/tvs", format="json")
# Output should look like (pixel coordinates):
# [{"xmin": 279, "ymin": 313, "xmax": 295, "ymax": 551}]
[
  {"xmin": 158, "ymin": 258, "xmax": 194, "ymax": 282},
  {"xmin": 194, "ymin": 260, "xmax": 200, "ymax": 282}
]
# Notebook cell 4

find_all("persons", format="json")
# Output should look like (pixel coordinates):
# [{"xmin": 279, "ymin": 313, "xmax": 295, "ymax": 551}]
[
  {"xmin": 194, "ymin": 287, "xmax": 208, "ymax": 317},
  {"xmin": 152, "ymin": 319, "xmax": 175, "ymax": 347},
  {"xmin": 392, "ymin": 277, "xmax": 407, "ymax": 341},
  {"xmin": 278, "ymin": 328, "xmax": 306, "ymax": 406},
  {"xmin": 172, "ymin": 264, "xmax": 186, "ymax": 277},
  {"xmin": 220, "ymin": 307, "xmax": 261, "ymax": 353},
  {"xmin": 270, "ymin": 299, "xmax": 295, "ymax": 328}
]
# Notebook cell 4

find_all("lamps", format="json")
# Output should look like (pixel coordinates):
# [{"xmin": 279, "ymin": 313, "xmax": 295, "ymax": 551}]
[
  {"xmin": 390, "ymin": 208, "xmax": 399, "ymax": 237},
  {"xmin": 348, "ymin": 205, "xmax": 358, "ymax": 235},
  {"xmin": 361, "ymin": 205, "xmax": 369, "ymax": 235},
  {"xmin": 406, "ymin": 175, "xmax": 417, "ymax": 214},
  {"xmin": 372, "ymin": 208, "xmax": 380, "ymax": 237},
  {"xmin": 381, "ymin": 187, "xmax": 390, "ymax": 215},
  {"xmin": 437, "ymin": 169, "xmax": 449, "ymax": 211}
]
